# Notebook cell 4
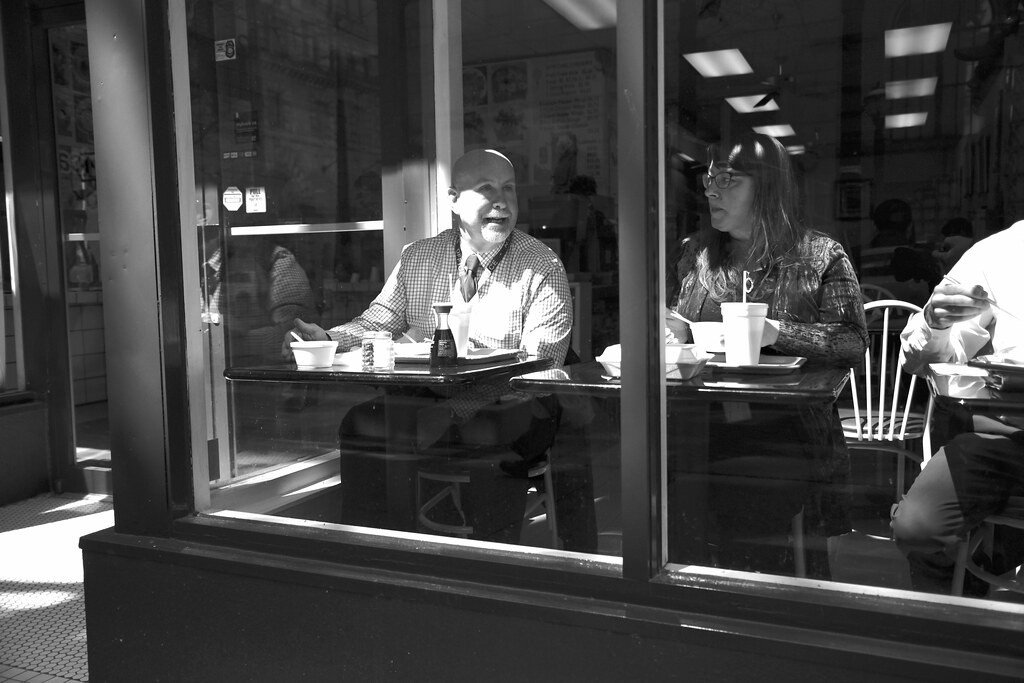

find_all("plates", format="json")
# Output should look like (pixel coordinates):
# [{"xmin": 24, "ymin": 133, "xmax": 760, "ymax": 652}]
[{"xmin": 359, "ymin": 342, "xmax": 473, "ymax": 355}]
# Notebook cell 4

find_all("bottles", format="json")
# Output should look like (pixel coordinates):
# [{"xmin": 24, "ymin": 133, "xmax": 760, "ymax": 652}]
[
  {"xmin": 360, "ymin": 331, "xmax": 374, "ymax": 371},
  {"xmin": 429, "ymin": 304, "xmax": 458, "ymax": 367},
  {"xmin": 374, "ymin": 332, "xmax": 395, "ymax": 371}
]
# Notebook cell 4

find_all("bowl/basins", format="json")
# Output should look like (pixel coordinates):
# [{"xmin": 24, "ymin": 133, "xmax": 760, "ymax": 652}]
[
  {"xmin": 966, "ymin": 356, "xmax": 1024, "ymax": 393},
  {"xmin": 289, "ymin": 340, "xmax": 339, "ymax": 367}
]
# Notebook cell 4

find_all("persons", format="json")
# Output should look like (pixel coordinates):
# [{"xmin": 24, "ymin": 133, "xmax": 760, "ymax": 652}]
[
  {"xmin": 199, "ymin": 196, "xmax": 318, "ymax": 458},
  {"xmin": 890, "ymin": 218, "xmax": 1024, "ymax": 603},
  {"xmin": 281, "ymin": 149, "xmax": 573, "ymax": 544},
  {"xmin": 665, "ymin": 134, "xmax": 869, "ymax": 582},
  {"xmin": 869, "ymin": 197, "xmax": 973, "ymax": 318},
  {"xmin": 530, "ymin": 168, "xmax": 618, "ymax": 314}
]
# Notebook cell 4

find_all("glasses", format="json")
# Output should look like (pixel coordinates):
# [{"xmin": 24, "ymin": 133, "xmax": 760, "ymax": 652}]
[{"xmin": 701, "ymin": 171, "xmax": 754, "ymax": 190}]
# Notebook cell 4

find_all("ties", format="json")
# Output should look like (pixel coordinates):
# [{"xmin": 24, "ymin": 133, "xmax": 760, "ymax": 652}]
[{"xmin": 459, "ymin": 254, "xmax": 481, "ymax": 302}]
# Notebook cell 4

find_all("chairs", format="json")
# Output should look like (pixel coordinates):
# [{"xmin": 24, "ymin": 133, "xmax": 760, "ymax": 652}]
[
  {"xmin": 417, "ymin": 284, "xmax": 582, "ymax": 540},
  {"xmin": 835, "ymin": 285, "xmax": 1024, "ymax": 598}
]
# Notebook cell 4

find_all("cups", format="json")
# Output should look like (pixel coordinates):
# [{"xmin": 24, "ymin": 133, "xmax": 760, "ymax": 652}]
[
  {"xmin": 689, "ymin": 321, "xmax": 726, "ymax": 352},
  {"xmin": 720, "ymin": 301, "xmax": 769, "ymax": 364},
  {"xmin": 434, "ymin": 303, "xmax": 472, "ymax": 357}
]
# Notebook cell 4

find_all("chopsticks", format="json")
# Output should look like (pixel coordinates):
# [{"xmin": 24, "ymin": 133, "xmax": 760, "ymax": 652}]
[
  {"xmin": 670, "ymin": 308, "xmax": 697, "ymax": 326},
  {"xmin": 943, "ymin": 274, "xmax": 1024, "ymax": 325}
]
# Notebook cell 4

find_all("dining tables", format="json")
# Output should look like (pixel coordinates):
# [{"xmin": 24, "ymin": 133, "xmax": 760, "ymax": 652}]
[
  {"xmin": 224, "ymin": 346, "xmax": 554, "ymax": 534},
  {"xmin": 510, "ymin": 350, "xmax": 851, "ymax": 577},
  {"xmin": 926, "ymin": 380, "xmax": 1024, "ymax": 610}
]
